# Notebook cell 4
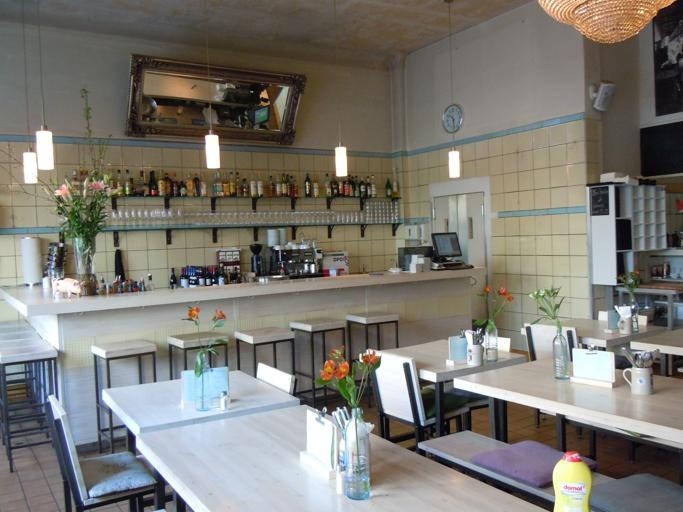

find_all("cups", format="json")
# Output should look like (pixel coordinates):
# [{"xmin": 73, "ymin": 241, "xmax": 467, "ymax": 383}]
[
  {"xmin": 466, "ymin": 343, "xmax": 485, "ymax": 367},
  {"xmin": 615, "ymin": 317, "xmax": 632, "ymax": 335},
  {"xmin": 42, "ymin": 276, "xmax": 51, "ymax": 288},
  {"xmin": 623, "ymin": 366, "xmax": 655, "ymax": 395}
]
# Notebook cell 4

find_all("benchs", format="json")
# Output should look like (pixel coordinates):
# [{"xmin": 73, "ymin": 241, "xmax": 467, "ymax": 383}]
[{"xmin": 417, "ymin": 429, "xmax": 615, "ymax": 512}]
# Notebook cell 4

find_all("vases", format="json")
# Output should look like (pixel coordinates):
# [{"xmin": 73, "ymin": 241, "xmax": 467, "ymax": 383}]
[{"xmin": 73, "ymin": 238, "xmax": 95, "ymax": 282}]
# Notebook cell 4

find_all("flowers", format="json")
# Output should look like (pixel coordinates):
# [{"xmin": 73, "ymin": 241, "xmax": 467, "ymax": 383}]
[
  {"xmin": 619, "ymin": 270, "xmax": 641, "ymax": 293},
  {"xmin": 27, "ymin": 88, "xmax": 119, "ymax": 239},
  {"xmin": 474, "ymin": 286, "xmax": 514, "ymax": 333}
]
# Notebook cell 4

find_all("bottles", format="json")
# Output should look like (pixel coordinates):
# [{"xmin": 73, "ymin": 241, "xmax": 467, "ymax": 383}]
[
  {"xmin": 551, "ymin": 328, "xmax": 570, "ymax": 379},
  {"xmin": 108, "ymin": 168, "xmax": 377, "ymax": 200},
  {"xmin": 392, "ymin": 176, "xmax": 400, "ymax": 197},
  {"xmin": 91, "ymin": 241, "xmax": 324, "ymax": 297},
  {"xmin": 219, "ymin": 391, "xmax": 231, "ymax": 411},
  {"xmin": 485, "ymin": 319, "xmax": 499, "ymax": 362},
  {"xmin": 193, "ymin": 351, "xmax": 210, "ymax": 411},
  {"xmin": 385, "ymin": 177, "xmax": 392, "ymax": 198}
]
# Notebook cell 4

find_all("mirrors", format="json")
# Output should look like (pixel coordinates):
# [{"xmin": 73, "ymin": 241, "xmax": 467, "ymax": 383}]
[{"xmin": 125, "ymin": 52, "xmax": 309, "ymax": 146}]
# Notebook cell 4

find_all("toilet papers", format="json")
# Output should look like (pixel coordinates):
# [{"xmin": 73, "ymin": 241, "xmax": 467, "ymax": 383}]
[{"xmin": 19, "ymin": 238, "xmax": 44, "ymax": 285}]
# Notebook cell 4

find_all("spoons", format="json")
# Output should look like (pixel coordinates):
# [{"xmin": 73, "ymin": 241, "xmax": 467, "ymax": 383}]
[
  {"xmin": 463, "ymin": 327, "xmax": 485, "ymax": 345},
  {"xmin": 620, "ymin": 345, "xmax": 660, "ymax": 368}
]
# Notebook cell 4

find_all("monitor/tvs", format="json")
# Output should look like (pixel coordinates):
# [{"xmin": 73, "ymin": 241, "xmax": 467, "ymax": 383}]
[
  {"xmin": 398, "ymin": 246, "xmax": 433, "ymax": 270},
  {"xmin": 253, "ymin": 106, "xmax": 270, "ymax": 125},
  {"xmin": 431, "ymin": 232, "xmax": 462, "ymax": 258}
]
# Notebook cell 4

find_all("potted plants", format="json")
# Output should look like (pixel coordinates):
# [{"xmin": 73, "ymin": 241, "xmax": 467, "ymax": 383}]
[{"xmin": 530, "ymin": 287, "xmax": 569, "ymax": 379}]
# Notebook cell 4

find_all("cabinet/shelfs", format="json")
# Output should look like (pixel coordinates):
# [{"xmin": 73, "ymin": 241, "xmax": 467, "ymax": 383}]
[
  {"xmin": 0, "ymin": 195, "xmax": 403, "ymax": 248},
  {"xmin": 587, "ymin": 183, "xmax": 667, "ymax": 286}
]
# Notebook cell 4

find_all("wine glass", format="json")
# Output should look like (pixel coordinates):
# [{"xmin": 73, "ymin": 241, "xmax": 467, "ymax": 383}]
[{"xmin": 105, "ymin": 200, "xmax": 400, "ymax": 228}]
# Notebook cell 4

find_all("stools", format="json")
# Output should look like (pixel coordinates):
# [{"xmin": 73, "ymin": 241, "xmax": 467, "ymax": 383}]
[
  {"xmin": 236, "ymin": 325, "xmax": 298, "ymax": 396},
  {"xmin": 346, "ymin": 308, "xmax": 399, "ymax": 377},
  {"xmin": 92, "ymin": 339, "xmax": 158, "ymax": 454},
  {"xmin": 0, "ymin": 320, "xmax": 60, "ymax": 473},
  {"xmin": 167, "ymin": 330, "xmax": 229, "ymax": 378},
  {"xmin": 289, "ymin": 317, "xmax": 347, "ymax": 409}
]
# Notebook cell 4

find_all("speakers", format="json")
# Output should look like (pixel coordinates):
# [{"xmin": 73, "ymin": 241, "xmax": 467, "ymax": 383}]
[{"xmin": 592, "ymin": 82, "xmax": 618, "ymax": 112}]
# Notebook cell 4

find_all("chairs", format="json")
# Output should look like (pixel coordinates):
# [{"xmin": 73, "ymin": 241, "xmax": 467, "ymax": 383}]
[
  {"xmin": 49, "ymin": 394, "xmax": 158, "ymax": 512},
  {"xmin": 372, "ymin": 309, "xmax": 648, "ymax": 451}
]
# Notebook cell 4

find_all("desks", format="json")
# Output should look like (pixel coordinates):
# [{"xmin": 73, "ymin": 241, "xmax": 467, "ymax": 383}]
[
  {"xmin": 616, "ymin": 280, "xmax": 683, "ymax": 330},
  {"xmin": 631, "ymin": 328, "xmax": 683, "ymax": 376},
  {"xmin": 136, "ymin": 404, "xmax": 551, "ymax": 512}
]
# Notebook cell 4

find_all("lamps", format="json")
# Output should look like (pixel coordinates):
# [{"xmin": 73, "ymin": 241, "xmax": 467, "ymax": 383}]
[
  {"xmin": 537, "ymin": 0, "xmax": 677, "ymax": 44},
  {"xmin": 203, "ymin": 0, "xmax": 222, "ymax": 169},
  {"xmin": 35, "ymin": 0, "xmax": 55, "ymax": 171},
  {"xmin": 335, "ymin": 0, "xmax": 348, "ymax": 176},
  {"xmin": 444, "ymin": 0, "xmax": 460, "ymax": 178},
  {"xmin": 590, "ymin": 82, "xmax": 617, "ymax": 111},
  {"xmin": 22, "ymin": 0, "xmax": 38, "ymax": 184}
]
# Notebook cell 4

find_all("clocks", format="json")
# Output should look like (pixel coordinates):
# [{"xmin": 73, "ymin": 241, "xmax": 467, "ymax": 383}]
[{"xmin": 442, "ymin": 103, "xmax": 464, "ymax": 134}]
